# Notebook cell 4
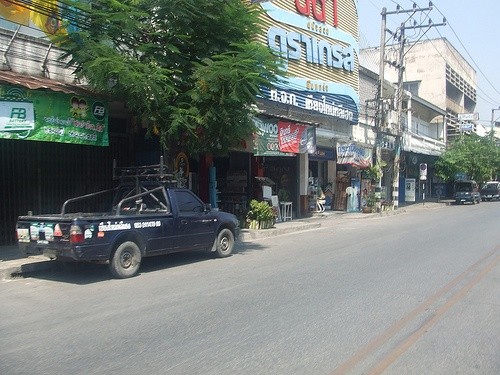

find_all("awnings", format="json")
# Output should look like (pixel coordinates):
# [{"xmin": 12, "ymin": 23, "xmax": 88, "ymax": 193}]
[{"xmin": 402, "ymin": 145, "xmax": 441, "ymax": 162}]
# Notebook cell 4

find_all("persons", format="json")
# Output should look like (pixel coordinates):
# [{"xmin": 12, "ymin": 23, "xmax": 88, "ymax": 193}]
[{"xmin": 317, "ymin": 187, "xmax": 327, "ymax": 212}]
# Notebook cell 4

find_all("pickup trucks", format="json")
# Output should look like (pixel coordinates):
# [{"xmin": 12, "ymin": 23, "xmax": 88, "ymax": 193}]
[
  {"xmin": 453, "ymin": 179, "xmax": 481, "ymax": 205},
  {"xmin": 14, "ymin": 189, "xmax": 239, "ymax": 279}
]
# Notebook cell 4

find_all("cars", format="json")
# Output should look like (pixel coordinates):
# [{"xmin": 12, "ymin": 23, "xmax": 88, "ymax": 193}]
[{"xmin": 480, "ymin": 181, "xmax": 500, "ymax": 202}]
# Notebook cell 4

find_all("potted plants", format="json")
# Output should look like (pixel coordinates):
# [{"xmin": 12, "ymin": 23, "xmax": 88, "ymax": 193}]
[
  {"xmin": 364, "ymin": 197, "xmax": 393, "ymax": 212},
  {"xmin": 246, "ymin": 199, "xmax": 276, "ymax": 229}
]
